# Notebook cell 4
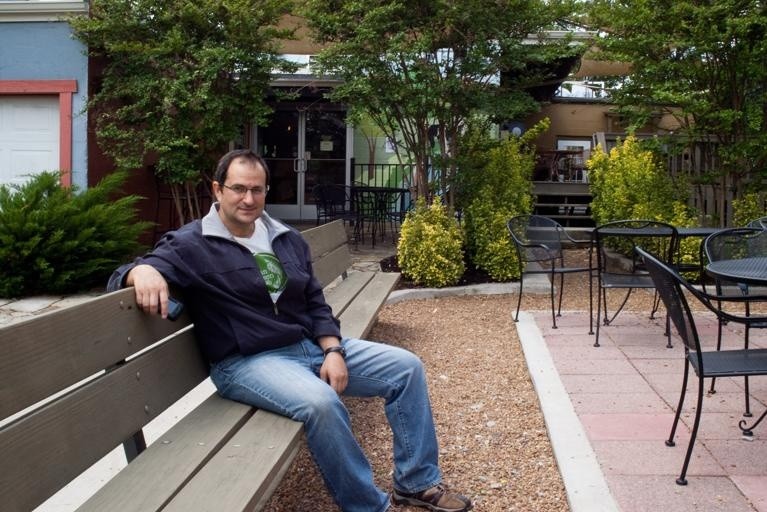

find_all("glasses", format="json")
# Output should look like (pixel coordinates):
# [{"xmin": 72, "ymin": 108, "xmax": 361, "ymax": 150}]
[{"xmin": 221, "ymin": 182, "xmax": 268, "ymax": 194}]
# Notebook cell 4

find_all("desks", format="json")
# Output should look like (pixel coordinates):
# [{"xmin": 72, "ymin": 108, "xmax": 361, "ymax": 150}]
[
  {"xmin": 704, "ymin": 256, "xmax": 767, "ymax": 287},
  {"xmin": 593, "ymin": 224, "xmax": 755, "ymax": 327}
]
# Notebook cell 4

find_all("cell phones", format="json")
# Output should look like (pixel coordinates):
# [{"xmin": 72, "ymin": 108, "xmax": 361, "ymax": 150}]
[{"xmin": 158, "ymin": 296, "xmax": 183, "ymax": 321}]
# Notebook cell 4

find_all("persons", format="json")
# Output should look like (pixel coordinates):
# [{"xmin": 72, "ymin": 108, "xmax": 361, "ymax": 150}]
[{"xmin": 107, "ymin": 148, "xmax": 473, "ymax": 511}]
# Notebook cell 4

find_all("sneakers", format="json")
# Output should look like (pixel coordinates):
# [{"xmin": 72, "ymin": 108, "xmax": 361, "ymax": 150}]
[{"xmin": 389, "ymin": 481, "xmax": 472, "ymax": 512}]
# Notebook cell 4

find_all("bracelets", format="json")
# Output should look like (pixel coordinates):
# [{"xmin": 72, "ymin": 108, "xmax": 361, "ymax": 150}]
[{"xmin": 324, "ymin": 346, "xmax": 346, "ymax": 357}]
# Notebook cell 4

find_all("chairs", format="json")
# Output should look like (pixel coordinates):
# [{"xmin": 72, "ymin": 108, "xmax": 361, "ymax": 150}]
[
  {"xmin": 632, "ymin": 244, "xmax": 766, "ymax": 486},
  {"xmin": 705, "ymin": 228, "xmax": 767, "ymax": 417},
  {"xmin": 314, "ymin": 176, "xmax": 409, "ymax": 251},
  {"xmin": 589, "ymin": 219, "xmax": 680, "ymax": 349},
  {"xmin": 507, "ymin": 214, "xmax": 598, "ymax": 331}
]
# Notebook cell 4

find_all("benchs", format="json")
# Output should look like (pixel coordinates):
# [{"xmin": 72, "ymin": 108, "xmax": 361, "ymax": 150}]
[{"xmin": 0, "ymin": 215, "xmax": 403, "ymax": 512}]
